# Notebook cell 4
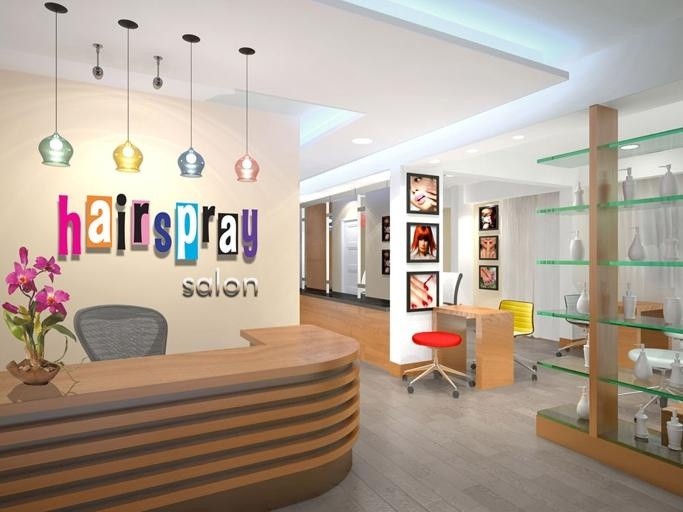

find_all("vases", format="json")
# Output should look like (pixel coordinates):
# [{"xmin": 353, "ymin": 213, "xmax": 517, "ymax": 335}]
[{"xmin": 4, "ymin": 357, "xmax": 61, "ymax": 386}]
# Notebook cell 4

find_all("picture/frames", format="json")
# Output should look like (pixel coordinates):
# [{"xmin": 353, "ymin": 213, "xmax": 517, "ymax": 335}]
[
  {"xmin": 479, "ymin": 204, "xmax": 499, "ymax": 231},
  {"xmin": 405, "ymin": 271, "xmax": 439, "ymax": 312},
  {"xmin": 478, "ymin": 235, "xmax": 499, "ymax": 260},
  {"xmin": 405, "ymin": 171, "xmax": 439, "ymax": 216},
  {"xmin": 381, "ymin": 249, "xmax": 391, "ymax": 275},
  {"xmin": 406, "ymin": 221, "xmax": 440, "ymax": 264},
  {"xmin": 382, "ymin": 216, "xmax": 390, "ymax": 242},
  {"xmin": 478, "ymin": 264, "xmax": 498, "ymax": 290}
]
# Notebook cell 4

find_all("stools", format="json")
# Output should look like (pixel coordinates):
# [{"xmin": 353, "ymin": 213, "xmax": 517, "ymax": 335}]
[
  {"xmin": 618, "ymin": 346, "xmax": 683, "ymax": 423},
  {"xmin": 403, "ymin": 331, "xmax": 476, "ymax": 400}
]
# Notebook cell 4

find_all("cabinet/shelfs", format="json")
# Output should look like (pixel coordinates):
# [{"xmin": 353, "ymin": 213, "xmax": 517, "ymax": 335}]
[
  {"xmin": 431, "ymin": 304, "xmax": 514, "ymax": 390},
  {"xmin": 532, "ymin": 101, "xmax": 683, "ymax": 496}
]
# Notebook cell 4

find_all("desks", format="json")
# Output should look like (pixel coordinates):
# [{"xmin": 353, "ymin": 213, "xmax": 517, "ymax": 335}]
[{"xmin": 618, "ymin": 298, "xmax": 672, "ymax": 369}]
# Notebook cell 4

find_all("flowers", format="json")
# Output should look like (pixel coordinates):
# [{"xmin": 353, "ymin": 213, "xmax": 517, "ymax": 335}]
[{"xmin": 1, "ymin": 246, "xmax": 78, "ymax": 363}]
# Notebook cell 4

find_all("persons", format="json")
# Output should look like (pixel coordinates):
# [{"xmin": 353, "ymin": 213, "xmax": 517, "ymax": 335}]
[
  {"xmin": 480, "ymin": 236, "xmax": 497, "ymax": 259},
  {"xmin": 480, "ymin": 267, "xmax": 492, "ymax": 285},
  {"xmin": 481, "ymin": 209, "xmax": 494, "ymax": 229},
  {"xmin": 410, "ymin": 177, "xmax": 437, "ymax": 212},
  {"xmin": 410, "ymin": 275, "xmax": 433, "ymax": 308},
  {"xmin": 410, "ymin": 224, "xmax": 437, "ymax": 259}
]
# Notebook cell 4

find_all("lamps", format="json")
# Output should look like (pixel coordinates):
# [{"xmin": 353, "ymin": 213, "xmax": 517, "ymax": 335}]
[
  {"xmin": 235, "ymin": 45, "xmax": 261, "ymax": 183},
  {"xmin": 179, "ymin": 33, "xmax": 206, "ymax": 178},
  {"xmin": 111, "ymin": 16, "xmax": 143, "ymax": 173},
  {"xmin": 38, "ymin": 1, "xmax": 73, "ymax": 168}
]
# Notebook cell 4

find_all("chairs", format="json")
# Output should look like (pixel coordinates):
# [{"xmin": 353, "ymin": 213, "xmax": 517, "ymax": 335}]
[
  {"xmin": 470, "ymin": 299, "xmax": 538, "ymax": 382},
  {"xmin": 73, "ymin": 304, "xmax": 169, "ymax": 362},
  {"xmin": 555, "ymin": 293, "xmax": 587, "ymax": 357}
]
